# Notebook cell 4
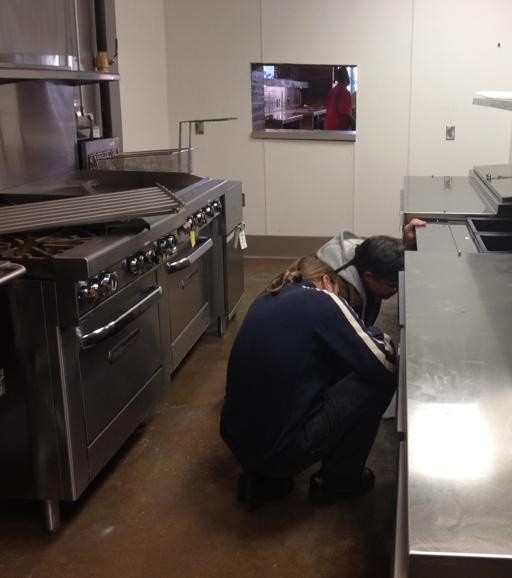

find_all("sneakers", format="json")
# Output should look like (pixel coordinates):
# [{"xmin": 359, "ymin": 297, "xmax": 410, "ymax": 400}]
[
  {"xmin": 241, "ymin": 474, "xmax": 282, "ymax": 511},
  {"xmin": 307, "ymin": 464, "xmax": 377, "ymax": 507}
]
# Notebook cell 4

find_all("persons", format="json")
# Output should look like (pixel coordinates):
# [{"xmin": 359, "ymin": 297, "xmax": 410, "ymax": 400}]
[
  {"xmin": 306, "ymin": 215, "xmax": 432, "ymax": 328},
  {"xmin": 324, "ymin": 67, "xmax": 354, "ymax": 131},
  {"xmin": 214, "ymin": 252, "xmax": 400, "ymax": 512}
]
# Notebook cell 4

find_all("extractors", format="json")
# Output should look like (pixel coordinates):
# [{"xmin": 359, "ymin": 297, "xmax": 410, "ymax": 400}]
[{"xmin": 0, "ymin": 63, "xmax": 120, "ymax": 86}]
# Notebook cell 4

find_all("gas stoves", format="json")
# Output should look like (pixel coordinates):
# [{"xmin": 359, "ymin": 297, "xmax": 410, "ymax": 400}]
[{"xmin": 0, "ymin": 227, "xmax": 92, "ymax": 256}]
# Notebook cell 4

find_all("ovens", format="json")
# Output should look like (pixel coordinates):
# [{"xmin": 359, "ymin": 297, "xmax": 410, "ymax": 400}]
[{"xmin": 63, "ymin": 229, "xmax": 214, "ymax": 500}]
